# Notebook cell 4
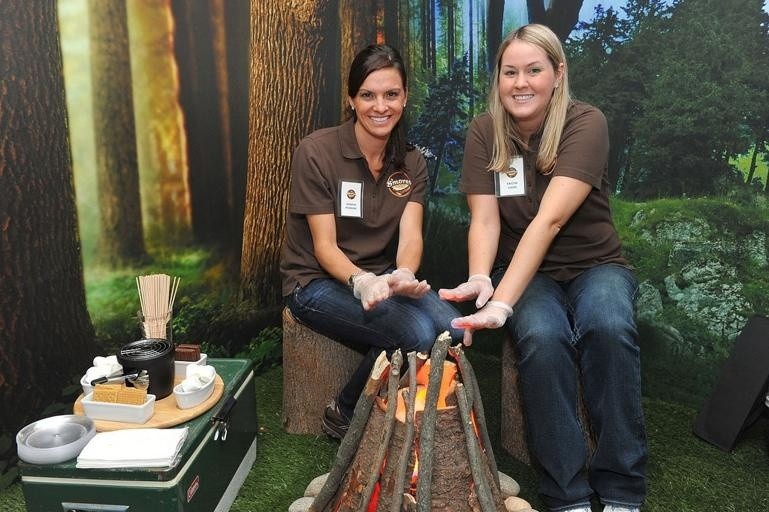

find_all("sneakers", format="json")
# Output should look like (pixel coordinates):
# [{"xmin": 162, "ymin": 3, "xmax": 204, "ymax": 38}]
[
  {"xmin": 603, "ymin": 504, "xmax": 640, "ymax": 512},
  {"xmin": 558, "ymin": 505, "xmax": 593, "ymax": 511},
  {"xmin": 321, "ymin": 399, "xmax": 352, "ymax": 442}
]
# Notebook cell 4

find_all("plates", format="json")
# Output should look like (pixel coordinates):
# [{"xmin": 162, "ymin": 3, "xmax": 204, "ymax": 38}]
[{"xmin": 16, "ymin": 415, "xmax": 96, "ymax": 465}]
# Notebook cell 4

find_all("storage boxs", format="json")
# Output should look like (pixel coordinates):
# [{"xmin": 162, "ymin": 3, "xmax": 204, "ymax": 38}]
[{"xmin": 18, "ymin": 358, "xmax": 261, "ymax": 512}]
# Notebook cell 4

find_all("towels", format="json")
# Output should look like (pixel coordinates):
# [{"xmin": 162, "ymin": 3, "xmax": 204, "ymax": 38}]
[{"xmin": 76, "ymin": 427, "xmax": 189, "ymax": 472}]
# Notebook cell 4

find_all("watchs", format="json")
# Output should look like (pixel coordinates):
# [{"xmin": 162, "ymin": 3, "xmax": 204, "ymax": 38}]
[{"xmin": 347, "ymin": 268, "xmax": 367, "ymax": 290}]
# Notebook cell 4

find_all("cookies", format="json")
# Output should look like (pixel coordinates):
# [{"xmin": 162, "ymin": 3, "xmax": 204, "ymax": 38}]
[{"xmin": 93, "ymin": 384, "xmax": 147, "ymax": 405}]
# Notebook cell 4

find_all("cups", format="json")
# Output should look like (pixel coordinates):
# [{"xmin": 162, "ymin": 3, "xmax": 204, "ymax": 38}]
[{"xmin": 137, "ymin": 309, "xmax": 174, "ymax": 342}]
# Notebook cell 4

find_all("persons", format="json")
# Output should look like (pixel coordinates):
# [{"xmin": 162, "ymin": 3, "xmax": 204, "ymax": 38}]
[
  {"xmin": 277, "ymin": 43, "xmax": 472, "ymax": 444},
  {"xmin": 438, "ymin": 23, "xmax": 648, "ymax": 512}
]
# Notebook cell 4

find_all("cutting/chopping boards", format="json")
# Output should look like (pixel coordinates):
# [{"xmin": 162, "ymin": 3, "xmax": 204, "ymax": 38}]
[{"xmin": 71, "ymin": 367, "xmax": 223, "ymax": 433}]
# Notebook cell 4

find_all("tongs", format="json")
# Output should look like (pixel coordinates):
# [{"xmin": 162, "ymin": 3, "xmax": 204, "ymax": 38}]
[{"xmin": 91, "ymin": 367, "xmax": 138, "ymax": 386}]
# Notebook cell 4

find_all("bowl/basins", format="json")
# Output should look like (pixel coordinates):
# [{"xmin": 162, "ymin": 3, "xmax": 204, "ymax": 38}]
[
  {"xmin": 172, "ymin": 365, "xmax": 220, "ymax": 411},
  {"xmin": 171, "ymin": 354, "xmax": 208, "ymax": 379},
  {"xmin": 80, "ymin": 370, "xmax": 125, "ymax": 394},
  {"xmin": 80, "ymin": 390, "xmax": 159, "ymax": 425}
]
